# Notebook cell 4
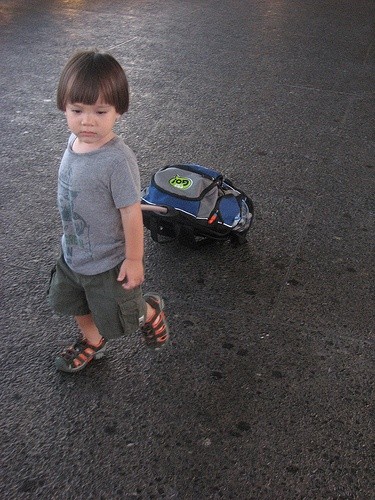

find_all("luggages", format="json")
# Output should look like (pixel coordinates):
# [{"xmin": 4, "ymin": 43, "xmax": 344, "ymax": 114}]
[{"xmin": 141, "ymin": 163, "xmax": 254, "ymax": 251}]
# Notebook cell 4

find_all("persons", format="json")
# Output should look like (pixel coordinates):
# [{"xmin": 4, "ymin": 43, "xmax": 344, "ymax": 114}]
[{"xmin": 47, "ymin": 51, "xmax": 170, "ymax": 373}]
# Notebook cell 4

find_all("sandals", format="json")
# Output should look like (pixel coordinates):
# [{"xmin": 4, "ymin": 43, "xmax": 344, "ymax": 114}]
[
  {"xmin": 54, "ymin": 332, "xmax": 107, "ymax": 372},
  {"xmin": 140, "ymin": 292, "xmax": 170, "ymax": 351}
]
